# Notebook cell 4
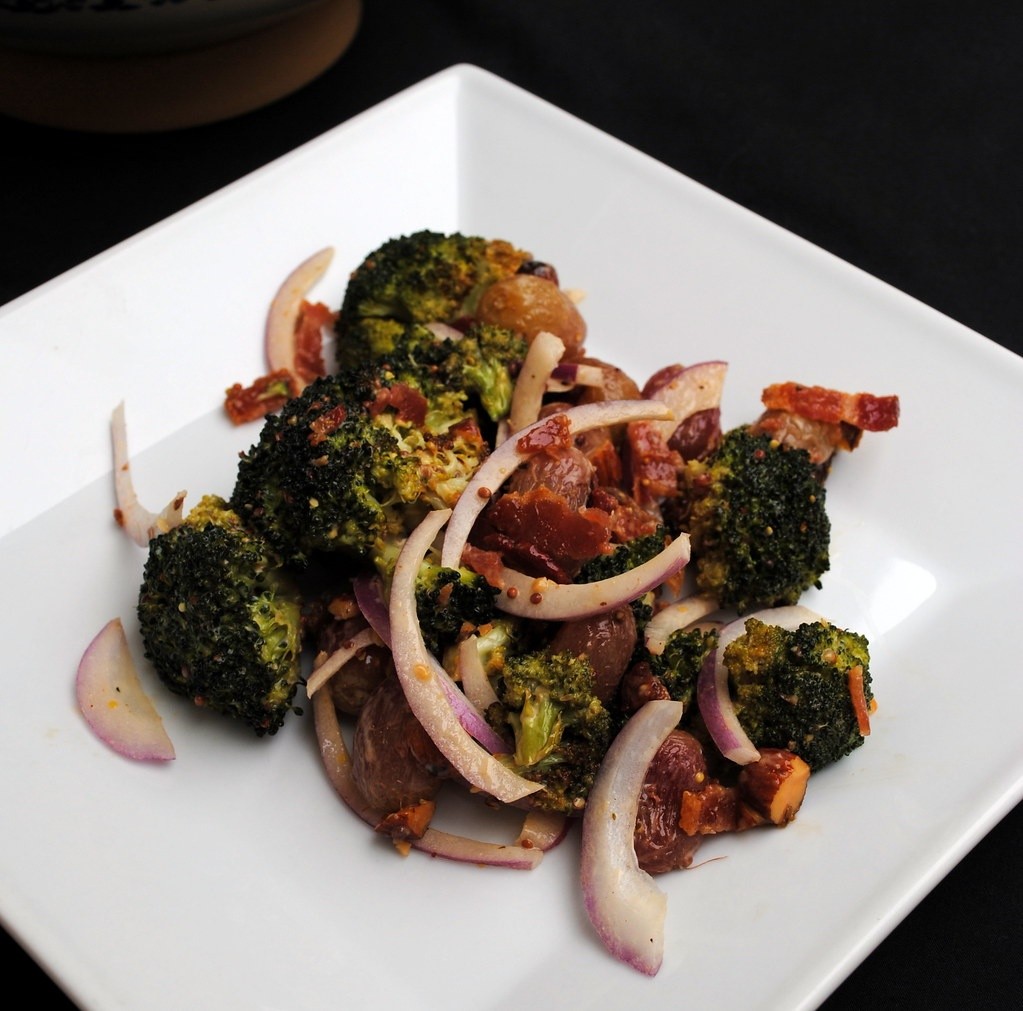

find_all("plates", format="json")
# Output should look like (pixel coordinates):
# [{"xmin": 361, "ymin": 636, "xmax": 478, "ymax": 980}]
[{"xmin": 0, "ymin": 62, "xmax": 1023, "ymax": 1011}]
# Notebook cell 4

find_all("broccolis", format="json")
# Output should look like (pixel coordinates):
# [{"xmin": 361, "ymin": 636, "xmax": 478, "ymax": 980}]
[
  {"xmin": 372, "ymin": 535, "xmax": 502, "ymax": 654},
  {"xmin": 572, "ymin": 528, "xmax": 667, "ymax": 622},
  {"xmin": 137, "ymin": 496, "xmax": 308, "ymax": 737},
  {"xmin": 650, "ymin": 618, "xmax": 874, "ymax": 775},
  {"xmin": 670, "ymin": 433, "xmax": 832, "ymax": 607},
  {"xmin": 229, "ymin": 371, "xmax": 421, "ymax": 588},
  {"xmin": 335, "ymin": 232, "xmax": 533, "ymax": 417},
  {"xmin": 488, "ymin": 651, "xmax": 610, "ymax": 811}
]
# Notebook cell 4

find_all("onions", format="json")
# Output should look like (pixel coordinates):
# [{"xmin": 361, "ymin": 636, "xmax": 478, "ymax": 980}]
[
  {"xmin": 266, "ymin": 249, "xmax": 331, "ymax": 378},
  {"xmin": 312, "ymin": 651, "xmax": 544, "ymax": 869},
  {"xmin": 304, "ymin": 626, "xmax": 383, "ymax": 699},
  {"xmin": 698, "ymin": 608, "xmax": 824, "ymax": 766},
  {"xmin": 491, "ymin": 533, "xmax": 690, "ymax": 619},
  {"xmin": 648, "ymin": 360, "xmax": 726, "ymax": 438},
  {"xmin": 510, "ymin": 329, "xmax": 565, "ymax": 436},
  {"xmin": 581, "ymin": 702, "xmax": 683, "ymax": 978},
  {"xmin": 76, "ymin": 618, "xmax": 177, "ymax": 762},
  {"xmin": 645, "ymin": 591, "xmax": 719, "ymax": 655},
  {"xmin": 440, "ymin": 399, "xmax": 677, "ymax": 570},
  {"xmin": 111, "ymin": 400, "xmax": 185, "ymax": 547},
  {"xmin": 390, "ymin": 507, "xmax": 546, "ymax": 802}
]
